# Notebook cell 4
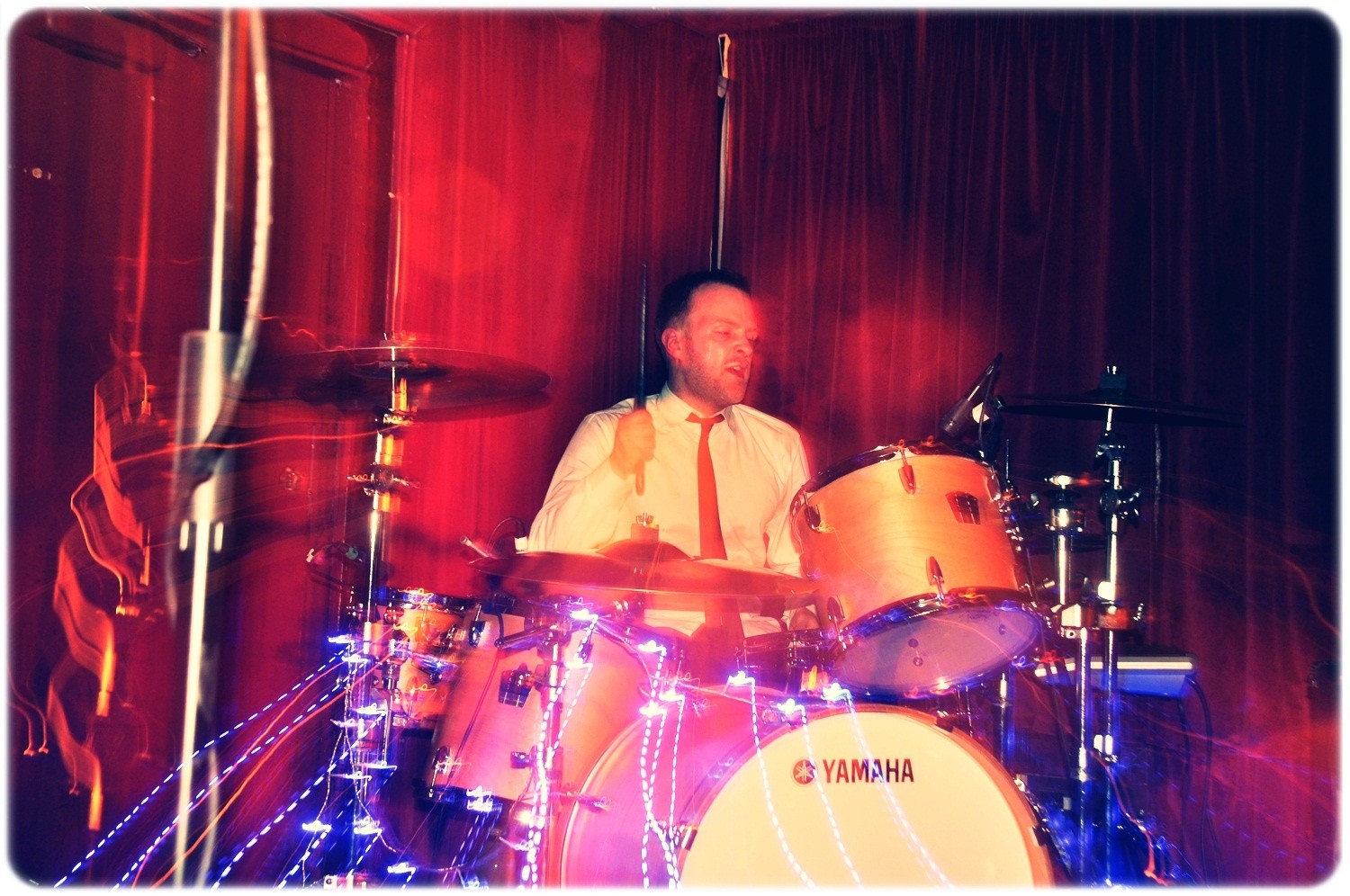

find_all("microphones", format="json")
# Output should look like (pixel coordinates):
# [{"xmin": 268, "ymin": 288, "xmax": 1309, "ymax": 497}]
[{"xmin": 938, "ymin": 351, "xmax": 1003, "ymax": 447}]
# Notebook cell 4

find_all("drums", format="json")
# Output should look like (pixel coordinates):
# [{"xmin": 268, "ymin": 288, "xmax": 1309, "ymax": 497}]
[
  {"xmin": 560, "ymin": 685, "xmax": 1057, "ymax": 890},
  {"xmin": 351, "ymin": 583, "xmax": 469, "ymax": 733},
  {"xmin": 427, "ymin": 594, "xmax": 688, "ymax": 821},
  {"xmin": 791, "ymin": 442, "xmax": 1048, "ymax": 704}
]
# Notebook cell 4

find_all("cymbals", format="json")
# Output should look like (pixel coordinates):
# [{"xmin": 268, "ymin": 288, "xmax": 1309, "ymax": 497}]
[
  {"xmin": 244, "ymin": 344, "xmax": 554, "ymax": 401},
  {"xmin": 468, "ymin": 541, "xmax": 818, "ymax": 598},
  {"xmin": 1005, "ymin": 387, "xmax": 1214, "ymax": 427}
]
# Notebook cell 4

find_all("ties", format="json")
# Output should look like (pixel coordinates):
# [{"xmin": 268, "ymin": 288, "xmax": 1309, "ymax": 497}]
[{"xmin": 685, "ymin": 414, "xmax": 726, "ymax": 560}]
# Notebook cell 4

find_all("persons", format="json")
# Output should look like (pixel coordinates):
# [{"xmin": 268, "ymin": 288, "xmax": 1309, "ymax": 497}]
[{"xmin": 515, "ymin": 269, "xmax": 821, "ymax": 639}]
